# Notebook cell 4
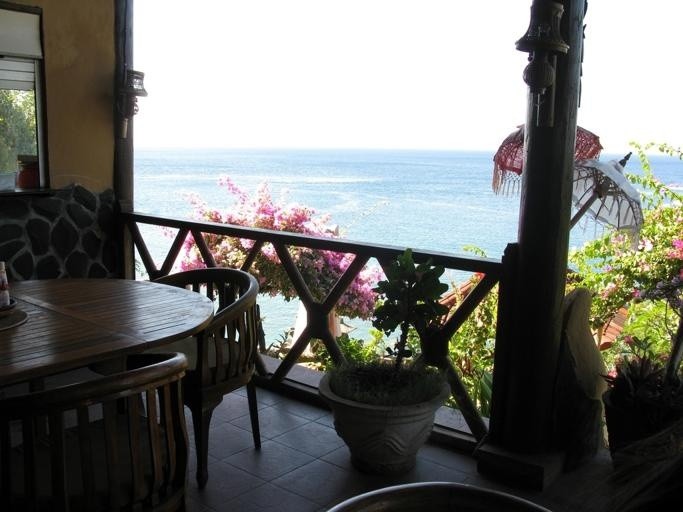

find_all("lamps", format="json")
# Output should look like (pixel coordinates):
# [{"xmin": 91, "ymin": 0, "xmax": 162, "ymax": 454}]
[
  {"xmin": 512, "ymin": 0, "xmax": 571, "ymax": 130},
  {"xmin": 114, "ymin": 66, "xmax": 147, "ymax": 141}
]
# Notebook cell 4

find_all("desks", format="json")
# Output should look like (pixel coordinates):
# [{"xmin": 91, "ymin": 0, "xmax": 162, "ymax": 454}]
[{"xmin": 3, "ymin": 278, "xmax": 216, "ymax": 442}]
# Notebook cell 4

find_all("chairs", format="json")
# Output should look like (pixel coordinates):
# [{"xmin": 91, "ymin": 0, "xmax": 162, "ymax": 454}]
[
  {"xmin": 1, "ymin": 350, "xmax": 192, "ymax": 510},
  {"xmin": 134, "ymin": 266, "xmax": 272, "ymax": 492}
]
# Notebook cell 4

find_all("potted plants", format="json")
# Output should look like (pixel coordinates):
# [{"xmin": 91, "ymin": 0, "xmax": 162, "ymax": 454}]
[
  {"xmin": 600, "ymin": 353, "xmax": 683, "ymax": 481},
  {"xmin": 315, "ymin": 247, "xmax": 453, "ymax": 477}
]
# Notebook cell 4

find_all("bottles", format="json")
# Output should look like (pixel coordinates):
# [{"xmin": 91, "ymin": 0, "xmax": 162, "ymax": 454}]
[{"xmin": 0, "ymin": 262, "xmax": 10, "ymax": 309}]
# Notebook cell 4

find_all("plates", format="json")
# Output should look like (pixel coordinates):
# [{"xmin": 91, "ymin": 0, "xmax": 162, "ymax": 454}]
[{"xmin": 0, "ymin": 310, "xmax": 28, "ymax": 332}]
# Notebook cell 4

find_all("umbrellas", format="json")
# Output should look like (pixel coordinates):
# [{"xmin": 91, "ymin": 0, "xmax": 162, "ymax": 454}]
[{"xmin": 494, "ymin": 120, "xmax": 645, "ymax": 233}]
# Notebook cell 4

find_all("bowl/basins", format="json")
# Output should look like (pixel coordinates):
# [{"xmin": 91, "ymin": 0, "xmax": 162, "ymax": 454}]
[{"xmin": 0, "ymin": 299, "xmax": 17, "ymax": 317}]
